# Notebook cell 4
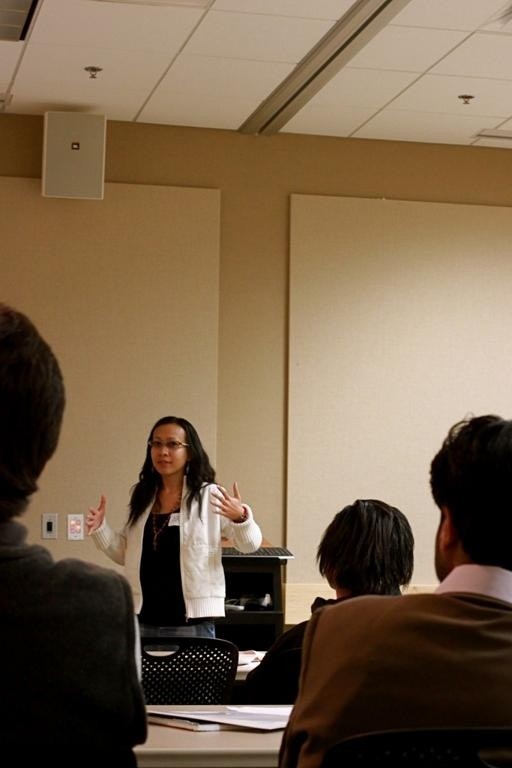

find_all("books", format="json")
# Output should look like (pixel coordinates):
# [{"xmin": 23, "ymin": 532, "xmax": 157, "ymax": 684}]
[{"xmin": 148, "ymin": 699, "xmax": 289, "ymax": 733}]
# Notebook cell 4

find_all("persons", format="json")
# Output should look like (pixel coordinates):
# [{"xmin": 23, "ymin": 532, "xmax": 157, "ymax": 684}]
[
  {"xmin": 2, "ymin": 304, "xmax": 143, "ymax": 767},
  {"xmin": 87, "ymin": 415, "xmax": 261, "ymax": 641}
]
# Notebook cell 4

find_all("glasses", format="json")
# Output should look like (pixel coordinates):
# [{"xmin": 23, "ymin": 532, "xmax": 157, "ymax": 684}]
[{"xmin": 147, "ymin": 439, "xmax": 189, "ymax": 449}]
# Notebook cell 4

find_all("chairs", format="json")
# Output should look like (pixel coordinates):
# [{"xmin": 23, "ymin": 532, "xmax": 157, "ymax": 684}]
[{"xmin": 140, "ymin": 638, "xmax": 239, "ymax": 705}]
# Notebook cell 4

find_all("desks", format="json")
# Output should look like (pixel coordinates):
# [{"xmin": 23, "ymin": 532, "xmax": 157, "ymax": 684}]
[{"xmin": 134, "ymin": 648, "xmax": 295, "ymax": 767}]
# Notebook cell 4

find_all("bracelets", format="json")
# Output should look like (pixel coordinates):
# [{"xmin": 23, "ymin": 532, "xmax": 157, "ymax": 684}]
[{"xmin": 236, "ymin": 507, "xmax": 248, "ymax": 522}]
